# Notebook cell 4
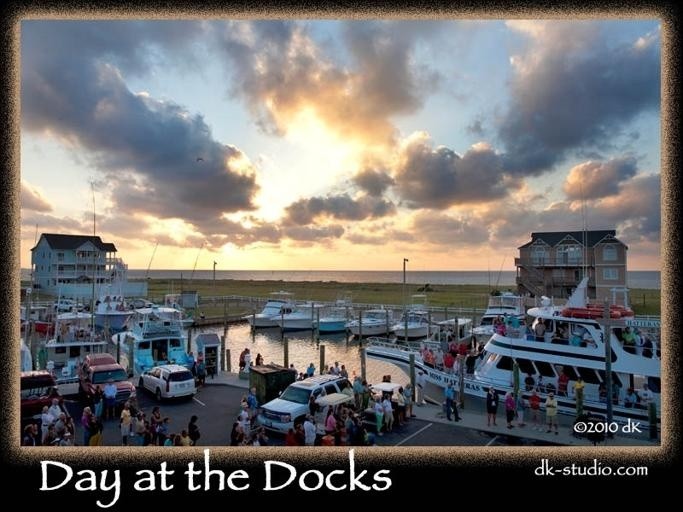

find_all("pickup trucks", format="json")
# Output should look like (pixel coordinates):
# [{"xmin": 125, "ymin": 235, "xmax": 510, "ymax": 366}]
[{"xmin": 20, "ymin": 369, "xmax": 75, "ymax": 441}]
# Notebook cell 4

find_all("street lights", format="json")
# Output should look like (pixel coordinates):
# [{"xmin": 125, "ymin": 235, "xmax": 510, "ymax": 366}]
[{"xmin": 402, "ymin": 256, "xmax": 409, "ymax": 303}]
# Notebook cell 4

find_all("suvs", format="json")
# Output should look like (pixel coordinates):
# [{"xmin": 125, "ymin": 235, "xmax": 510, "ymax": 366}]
[
  {"xmin": 137, "ymin": 362, "xmax": 197, "ymax": 404},
  {"xmin": 75, "ymin": 350, "xmax": 136, "ymax": 408},
  {"xmin": 254, "ymin": 368, "xmax": 352, "ymax": 436}
]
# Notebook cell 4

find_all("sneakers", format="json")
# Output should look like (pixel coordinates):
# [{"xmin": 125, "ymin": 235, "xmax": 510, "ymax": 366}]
[
  {"xmin": 506, "ymin": 425, "xmax": 515, "ymax": 429},
  {"xmin": 376, "ymin": 414, "xmax": 417, "ymax": 437},
  {"xmin": 447, "ymin": 417, "xmax": 462, "ymax": 423},
  {"xmin": 532, "ymin": 426, "xmax": 544, "ymax": 432},
  {"xmin": 518, "ymin": 423, "xmax": 526, "ymax": 426},
  {"xmin": 546, "ymin": 429, "xmax": 559, "ymax": 436},
  {"xmin": 487, "ymin": 423, "xmax": 497, "ymax": 427}
]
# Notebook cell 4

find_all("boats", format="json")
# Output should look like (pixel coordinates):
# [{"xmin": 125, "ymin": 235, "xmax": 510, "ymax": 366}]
[
  {"xmin": 240, "ymin": 286, "xmax": 536, "ymax": 341},
  {"xmin": 361, "ymin": 274, "xmax": 662, "ymax": 439}
]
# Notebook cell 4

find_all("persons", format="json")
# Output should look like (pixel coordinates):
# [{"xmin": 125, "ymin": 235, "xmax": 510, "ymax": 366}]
[
  {"xmin": 230, "ymin": 347, "xmax": 416, "ymax": 446},
  {"xmin": 23, "ymin": 349, "xmax": 210, "ymax": 446},
  {"xmin": 419, "ymin": 312, "xmax": 661, "ymax": 434}
]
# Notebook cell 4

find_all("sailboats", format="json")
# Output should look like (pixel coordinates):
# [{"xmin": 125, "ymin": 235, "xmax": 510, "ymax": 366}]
[{"xmin": 20, "ymin": 178, "xmax": 196, "ymax": 384}]
